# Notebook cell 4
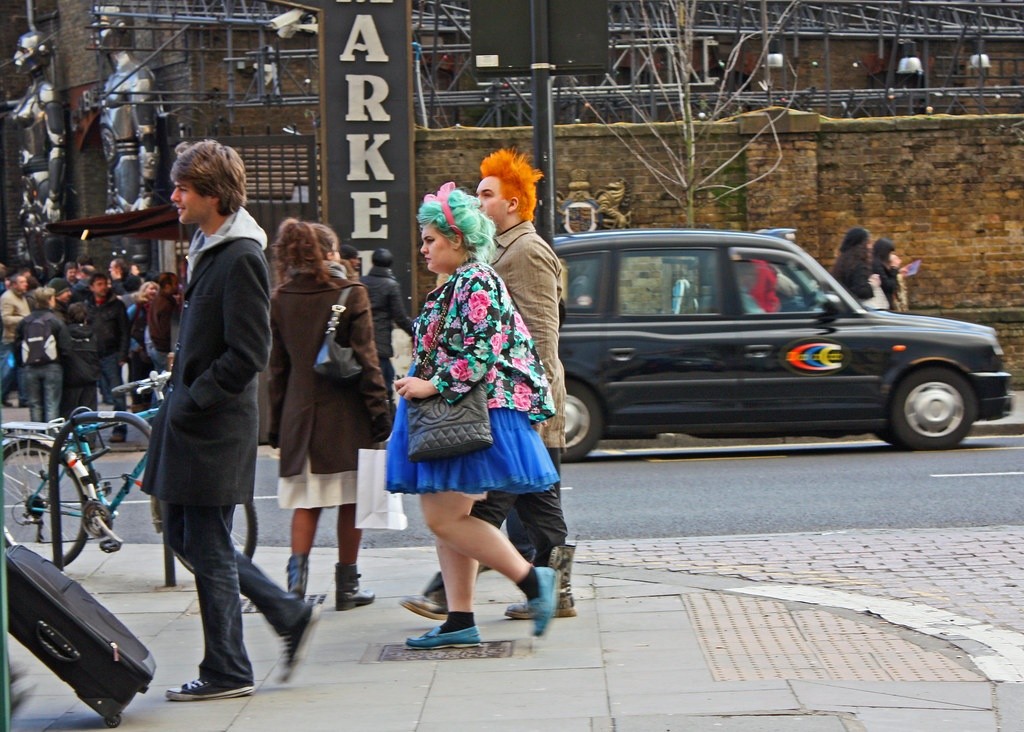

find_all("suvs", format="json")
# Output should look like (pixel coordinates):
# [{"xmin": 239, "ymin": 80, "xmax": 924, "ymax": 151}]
[{"xmin": 546, "ymin": 227, "xmax": 1016, "ymax": 463}]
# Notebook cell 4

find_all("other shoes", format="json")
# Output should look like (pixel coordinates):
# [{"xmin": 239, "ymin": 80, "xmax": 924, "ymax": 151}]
[
  {"xmin": 132, "ymin": 404, "xmax": 144, "ymax": 411},
  {"xmin": 90, "ymin": 442, "xmax": 95, "ymax": 448},
  {"xmin": 109, "ymin": 433, "xmax": 126, "ymax": 442}
]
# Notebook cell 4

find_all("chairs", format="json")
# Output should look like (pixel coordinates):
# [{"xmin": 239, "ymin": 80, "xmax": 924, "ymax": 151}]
[{"xmin": 571, "ymin": 276, "xmax": 587, "ymax": 299}]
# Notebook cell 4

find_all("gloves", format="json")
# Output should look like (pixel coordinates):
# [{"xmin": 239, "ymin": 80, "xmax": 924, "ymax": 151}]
[
  {"xmin": 267, "ymin": 432, "xmax": 279, "ymax": 449},
  {"xmin": 371, "ymin": 412, "xmax": 392, "ymax": 441}
]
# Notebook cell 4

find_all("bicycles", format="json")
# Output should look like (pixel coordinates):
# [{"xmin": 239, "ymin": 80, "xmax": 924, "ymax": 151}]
[{"xmin": 0, "ymin": 370, "xmax": 258, "ymax": 582}]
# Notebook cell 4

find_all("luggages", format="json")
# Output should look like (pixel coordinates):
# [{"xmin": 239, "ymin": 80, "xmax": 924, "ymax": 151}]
[{"xmin": 7, "ymin": 545, "xmax": 156, "ymax": 727}]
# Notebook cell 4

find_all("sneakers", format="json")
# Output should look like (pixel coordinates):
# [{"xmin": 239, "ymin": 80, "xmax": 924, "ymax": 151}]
[
  {"xmin": 528, "ymin": 567, "xmax": 556, "ymax": 635},
  {"xmin": 167, "ymin": 678, "xmax": 255, "ymax": 700},
  {"xmin": 405, "ymin": 625, "xmax": 482, "ymax": 649},
  {"xmin": 278, "ymin": 604, "xmax": 323, "ymax": 682}
]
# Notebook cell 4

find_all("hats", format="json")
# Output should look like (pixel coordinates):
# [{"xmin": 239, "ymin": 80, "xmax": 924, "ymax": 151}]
[{"xmin": 47, "ymin": 278, "xmax": 69, "ymax": 295}]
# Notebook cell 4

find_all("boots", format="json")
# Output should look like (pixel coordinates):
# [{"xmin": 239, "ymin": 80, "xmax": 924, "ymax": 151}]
[
  {"xmin": 336, "ymin": 564, "xmax": 376, "ymax": 610},
  {"xmin": 287, "ymin": 557, "xmax": 308, "ymax": 600},
  {"xmin": 398, "ymin": 572, "xmax": 449, "ymax": 620},
  {"xmin": 506, "ymin": 546, "xmax": 575, "ymax": 617}
]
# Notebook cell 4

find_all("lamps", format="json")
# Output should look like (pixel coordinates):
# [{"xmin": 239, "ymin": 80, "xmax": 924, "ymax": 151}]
[
  {"xmin": 966, "ymin": 38, "xmax": 992, "ymax": 69},
  {"xmin": 760, "ymin": 39, "xmax": 783, "ymax": 67},
  {"xmin": 895, "ymin": 38, "xmax": 924, "ymax": 74}
]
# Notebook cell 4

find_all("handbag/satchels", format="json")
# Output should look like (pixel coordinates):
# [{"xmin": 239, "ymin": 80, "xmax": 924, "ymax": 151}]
[
  {"xmin": 0, "ymin": 350, "xmax": 15, "ymax": 379},
  {"xmin": 313, "ymin": 285, "xmax": 362, "ymax": 388},
  {"xmin": 355, "ymin": 447, "xmax": 408, "ymax": 530},
  {"xmin": 849, "ymin": 274, "xmax": 890, "ymax": 309},
  {"xmin": 408, "ymin": 275, "xmax": 492, "ymax": 461}
]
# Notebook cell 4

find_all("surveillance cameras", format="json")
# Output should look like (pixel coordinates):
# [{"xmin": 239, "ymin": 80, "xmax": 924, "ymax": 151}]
[{"xmin": 271, "ymin": 8, "xmax": 308, "ymax": 29}]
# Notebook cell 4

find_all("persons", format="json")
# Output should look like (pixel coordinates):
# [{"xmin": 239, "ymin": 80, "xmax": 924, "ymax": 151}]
[
  {"xmin": 360, "ymin": 247, "xmax": 416, "ymax": 420},
  {"xmin": 86, "ymin": 12, "xmax": 161, "ymax": 271},
  {"xmin": 339, "ymin": 244, "xmax": 359, "ymax": 269},
  {"xmin": 0, "ymin": 257, "xmax": 182, "ymax": 453},
  {"xmin": 140, "ymin": 140, "xmax": 320, "ymax": 703},
  {"xmin": 267, "ymin": 216, "xmax": 393, "ymax": 612},
  {"xmin": 8, "ymin": 32, "xmax": 67, "ymax": 283},
  {"xmin": 401, "ymin": 148, "xmax": 579, "ymax": 620},
  {"xmin": 383, "ymin": 184, "xmax": 562, "ymax": 650},
  {"xmin": 731, "ymin": 228, "xmax": 801, "ymax": 312},
  {"xmin": 834, "ymin": 227, "xmax": 902, "ymax": 312}
]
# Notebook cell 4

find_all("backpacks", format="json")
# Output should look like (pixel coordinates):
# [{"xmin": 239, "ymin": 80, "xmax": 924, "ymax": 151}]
[
  {"xmin": 67, "ymin": 341, "xmax": 101, "ymax": 381},
  {"xmin": 20, "ymin": 313, "xmax": 58, "ymax": 365}
]
